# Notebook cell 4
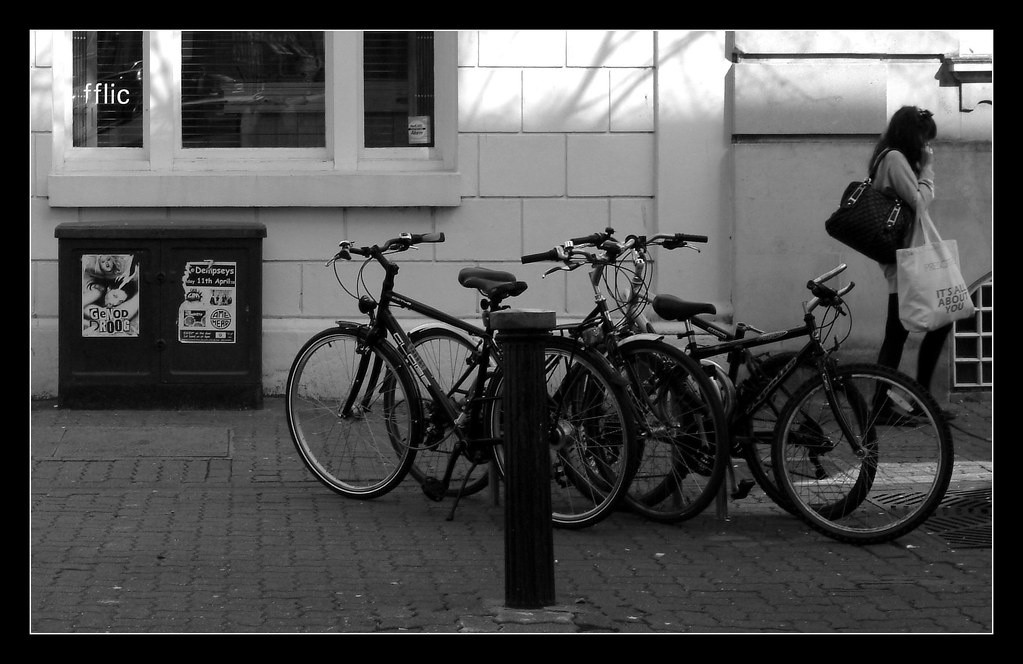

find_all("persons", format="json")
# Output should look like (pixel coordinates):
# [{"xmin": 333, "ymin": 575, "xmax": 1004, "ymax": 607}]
[
  {"xmin": 865, "ymin": 105, "xmax": 955, "ymax": 424},
  {"xmin": 82, "ymin": 254, "xmax": 139, "ymax": 336}
]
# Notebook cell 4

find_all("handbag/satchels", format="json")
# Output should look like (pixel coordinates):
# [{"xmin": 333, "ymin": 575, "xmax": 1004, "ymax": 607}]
[
  {"xmin": 896, "ymin": 208, "xmax": 975, "ymax": 331},
  {"xmin": 826, "ymin": 148, "xmax": 919, "ymax": 263}
]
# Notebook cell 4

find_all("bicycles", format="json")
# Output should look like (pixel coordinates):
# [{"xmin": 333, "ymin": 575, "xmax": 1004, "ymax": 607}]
[{"xmin": 285, "ymin": 227, "xmax": 954, "ymax": 545}]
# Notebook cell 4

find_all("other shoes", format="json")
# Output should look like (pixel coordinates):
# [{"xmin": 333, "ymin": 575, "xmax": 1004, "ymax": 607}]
[
  {"xmin": 909, "ymin": 398, "xmax": 956, "ymax": 423},
  {"xmin": 872, "ymin": 407, "xmax": 918, "ymax": 428}
]
